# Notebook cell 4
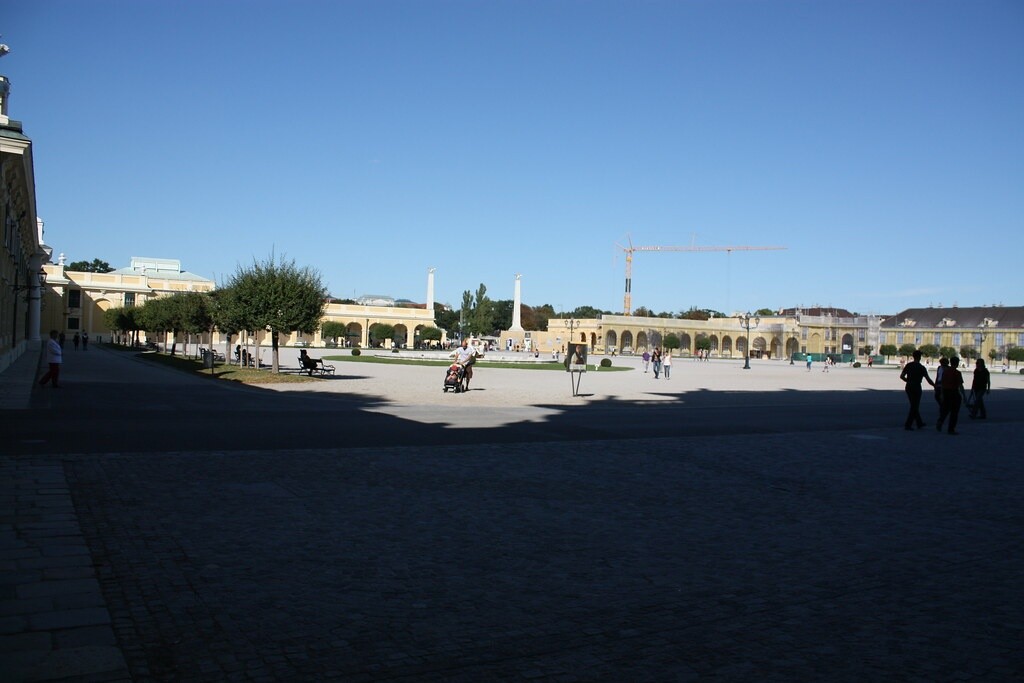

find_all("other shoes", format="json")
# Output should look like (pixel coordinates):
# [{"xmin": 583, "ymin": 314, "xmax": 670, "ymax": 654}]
[
  {"xmin": 917, "ymin": 422, "xmax": 926, "ymax": 428},
  {"xmin": 52, "ymin": 383, "xmax": 65, "ymax": 388},
  {"xmin": 948, "ymin": 432, "xmax": 959, "ymax": 435},
  {"xmin": 968, "ymin": 415, "xmax": 975, "ymax": 420},
  {"xmin": 39, "ymin": 382, "xmax": 48, "ymax": 389},
  {"xmin": 936, "ymin": 426, "xmax": 941, "ymax": 432},
  {"xmin": 977, "ymin": 416, "xmax": 986, "ymax": 419},
  {"xmin": 905, "ymin": 427, "xmax": 914, "ymax": 431}
]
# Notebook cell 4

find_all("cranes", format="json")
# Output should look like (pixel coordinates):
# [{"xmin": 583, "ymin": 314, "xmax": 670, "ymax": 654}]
[{"xmin": 612, "ymin": 232, "xmax": 789, "ymax": 316}]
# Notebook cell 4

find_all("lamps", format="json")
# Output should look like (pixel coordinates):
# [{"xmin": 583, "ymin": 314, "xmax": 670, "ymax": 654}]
[{"xmin": 9, "ymin": 268, "xmax": 48, "ymax": 311}]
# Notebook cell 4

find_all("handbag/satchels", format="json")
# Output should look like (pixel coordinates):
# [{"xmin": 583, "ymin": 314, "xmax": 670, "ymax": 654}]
[
  {"xmin": 466, "ymin": 356, "xmax": 476, "ymax": 367},
  {"xmin": 969, "ymin": 403, "xmax": 973, "ymax": 412}
]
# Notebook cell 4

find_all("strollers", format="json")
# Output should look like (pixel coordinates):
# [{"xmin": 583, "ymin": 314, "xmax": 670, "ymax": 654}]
[{"xmin": 442, "ymin": 356, "xmax": 469, "ymax": 393}]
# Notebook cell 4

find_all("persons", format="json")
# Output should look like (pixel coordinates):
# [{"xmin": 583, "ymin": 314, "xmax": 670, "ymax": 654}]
[
  {"xmin": 72, "ymin": 330, "xmax": 88, "ymax": 352},
  {"xmin": 806, "ymin": 353, "xmax": 812, "ymax": 370},
  {"xmin": 427, "ymin": 341, "xmax": 450, "ymax": 349},
  {"xmin": 571, "ymin": 344, "xmax": 585, "ymax": 364},
  {"xmin": 146, "ymin": 338, "xmax": 153, "ymax": 343},
  {"xmin": 643, "ymin": 350, "xmax": 650, "ymax": 373},
  {"xmin": 900, "ymin": 350, "xmax": 935, "ymax": 431},
  {"xmin": 552, "ymin": 349, "xmax": 567, "ymax": 359},
  {"xmin": 446, "ymin": 340, "xmax": 473, "ymax": 391},
  {"xmin": 968, "ymin": 358, "xmax": 990, "ymax": 419},
  {"xmin": 236, "ymin": 345, "xmax": 251, "ymax": 357},
  {"xmin": 934, "ymin": 357, "xmax": 967, "ymax": 435},
  {"xmin": 652, "ymin": 348, "xmax": 661, "ymax": 379},
  {"xmin": 39, "ymin": 330, "xmax": 65, "ymax": 388},
  {"xmin": 535, "ymin": 349, "xmax": 539, "ymax": 357},
  {"xmin": 611, "ymin": 347, "xmax": 616, "ymax": 357},
  {"xmin": 868, "ymin": 357, "xmax": 872, "ymax": 367},
  {"xmin": 822, "ymin": 357, "xmax": 833, "ymax": 373},
  {"xmin": 694, "ymin": 351, "xmax": 708, "ymax": 361},
  {"xmin": 301, "ymin": 350, "xmax": 317, "ymax": 375},
  {"xmin": 662, "ymin": 351, "xmax": 671, "ymax": 379}
]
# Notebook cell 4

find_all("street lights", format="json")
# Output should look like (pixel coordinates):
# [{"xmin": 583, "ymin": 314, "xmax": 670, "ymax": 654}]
[
  {"xmin": 564, "ymin": 316, "xmax": 580, "ymax": 342},
  {"xmin": 786, "ymin": 328, "xmax": 800, "ymax": 365},
  {"xmin": 738, "ymin": 311, "xmax": 760, "ymax": 369}
]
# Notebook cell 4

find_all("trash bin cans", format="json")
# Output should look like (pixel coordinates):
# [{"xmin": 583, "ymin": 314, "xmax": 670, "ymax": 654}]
[{"xmin": 203, "ymin": 349, "xmax": 215, "ymax": 369}]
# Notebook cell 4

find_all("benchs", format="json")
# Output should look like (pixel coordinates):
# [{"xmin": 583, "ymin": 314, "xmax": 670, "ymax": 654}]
[
  {"xmin": 235, "ymin": 352, "xmax": 256, "ymax": 367},
  {"xmin": 200, "ymin": 348, "xmax": 218, "ymax": 360},
  {"xmin": 299, "ymin": 358, "xmax": 335, "ymax": 378}
]
